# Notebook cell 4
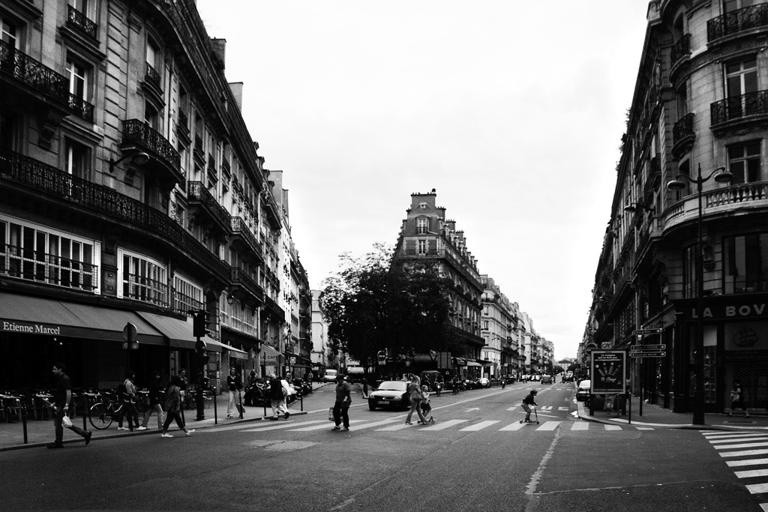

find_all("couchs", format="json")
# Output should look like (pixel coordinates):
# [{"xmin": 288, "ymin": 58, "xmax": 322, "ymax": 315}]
[{"xmin": 200, "ymin": 307, "xmax": 211, "ymax": 338}]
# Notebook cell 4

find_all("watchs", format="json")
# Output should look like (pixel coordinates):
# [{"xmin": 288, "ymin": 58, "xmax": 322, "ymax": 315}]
[
  {"xmin": 433, "ymin": 381, "xmax": 444, "ymax": 396},
  {"xmin": 501, "ymin": 380, "xmax": 506, "ymax": 389},
  {"xmin": 242, "ymin": 376, "xmax": 309, "ymax": 404}
]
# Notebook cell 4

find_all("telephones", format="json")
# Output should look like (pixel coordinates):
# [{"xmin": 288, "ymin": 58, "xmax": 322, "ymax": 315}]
[
  {"xmin": 629, "ymin": 351, "xmax": 667, "ymax": 358},
  {"xmin": 629, "ymin": 344, "xmax": 667, "ymax": 352},
  {"xmin": 630, "ymin": 328, "xmax": 664, "ymax": 335}
]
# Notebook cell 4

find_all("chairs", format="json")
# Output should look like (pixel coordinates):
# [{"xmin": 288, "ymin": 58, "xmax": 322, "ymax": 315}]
[
  {"xmin": 331, "ymin": 426, "xmax": 340, "ymax": 430},
  {"xmin": 82, "ymin": 431, "xmax": 91, "ymax": 444},
  {"xmin": 47, "ymin": 439, "xmax": 64, "ymax": 448},
  {"xmin": 118, "ymin": 425, "xmax": 151, "ymax": 430},
  {"xmin": 185, "ymin": 428, "xmax": 196, "ymax": 437},
  {"xmin": 162, "ymin": 433, "xmax": 173, "ymax": 438},
  {"xmin": 341, "ymin": 426, "xmax": 348, "ymax": 431}
]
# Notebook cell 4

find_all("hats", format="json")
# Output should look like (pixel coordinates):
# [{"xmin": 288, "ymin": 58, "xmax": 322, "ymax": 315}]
[
  {"xmin": 559, "ymin": 370, "xmax": 574, "ymax": 383},
  {"xmin": 518, "ymin": 372, "xmax": 553, "ymax": 384},
  {"xmin": 367, "ymin": 379, "xmax": 412, "ymax": 411},
  {"xmin": 575, "ymin": 380, "xmax": 591, "ymax": 401}
]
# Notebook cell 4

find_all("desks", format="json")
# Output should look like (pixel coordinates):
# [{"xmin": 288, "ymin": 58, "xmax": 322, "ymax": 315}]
[
  {"xmin": 666, "ymin": 162, "xmax": 735, "ymax": 424},
  {"xmin": 286, "ymin": 324, "xmax": 293, "ymax": 372}
]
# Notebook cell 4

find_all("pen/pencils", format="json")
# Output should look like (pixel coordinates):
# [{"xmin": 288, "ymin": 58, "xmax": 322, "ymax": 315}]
[{"xmin": 322, "ymin": 367, "xmax": 338, "ymax": 384}]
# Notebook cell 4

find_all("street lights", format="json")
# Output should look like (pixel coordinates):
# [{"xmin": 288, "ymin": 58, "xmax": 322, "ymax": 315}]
[
  {"xmin": 329, "ymin": 407, "xmax": 343, "ymax": 421},
  {"xmin": 412, "ymin": 393, "xmax": 422, "ymax": 401}
]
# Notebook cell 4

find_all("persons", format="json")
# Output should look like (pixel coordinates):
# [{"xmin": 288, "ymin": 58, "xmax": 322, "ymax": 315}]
[
  {"xmin": 269, "ymin": 372, "xmax": 289, "ymax": 420},
  {"xmin": 48, "ymin": 363, "xmax": 91, "ymax": 448},
  {"xmin": 331, "ymin": 374, "xmax": 352, "ymax": 432},
  {"xmin": 307, "ymin": 370, "xmax": 314, "ymax": 385},
  {"xmin": 247, "ymin": 370, "xmax": 257, "ymax": 405},
  {"xmin": 405, "ymin": 374, "xmax": 459, "ymax": 426},
  {"xmin": 282, "ymin": 373, "xmax": 300, "ymax": 406},
  {"xmin": 225, "ymin": 367, "xmax": 245, "ymax": 419},
  {"xmin": 727, "ymin": 379, "xmax": 750, "ymax": 418},
  {"xmin": 117, "ymin": 368, "xmax": 196, "ymax": 438},
  {"xmin": 520, "ymin": 390, "xmax": 538, "ymax": 422}
]
